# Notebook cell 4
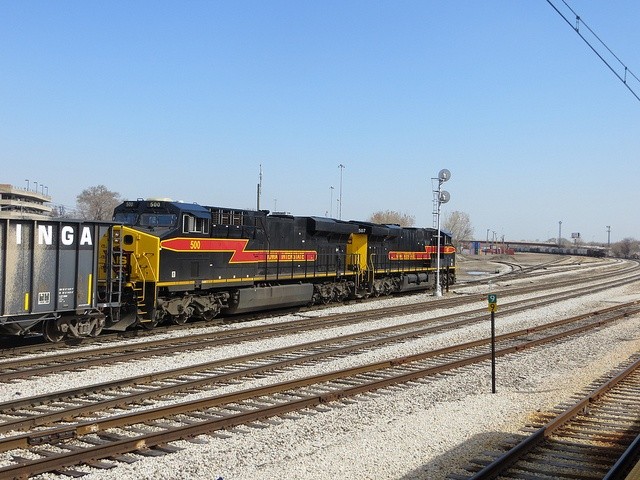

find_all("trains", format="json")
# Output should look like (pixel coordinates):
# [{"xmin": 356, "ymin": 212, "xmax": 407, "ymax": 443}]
[{"xmin": 0, "ymin": 198, "xmax": 456, "ymax": 343}]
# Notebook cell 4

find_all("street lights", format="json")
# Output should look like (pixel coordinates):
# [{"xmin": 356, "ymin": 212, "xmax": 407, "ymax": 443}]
[
  {"xmin": 338, "ymin": 163, "xmax": 345, "ymax": 219},
  {"xmin": 329, "ymin": 186, "xmax": 334, "ymax": 217}
]
